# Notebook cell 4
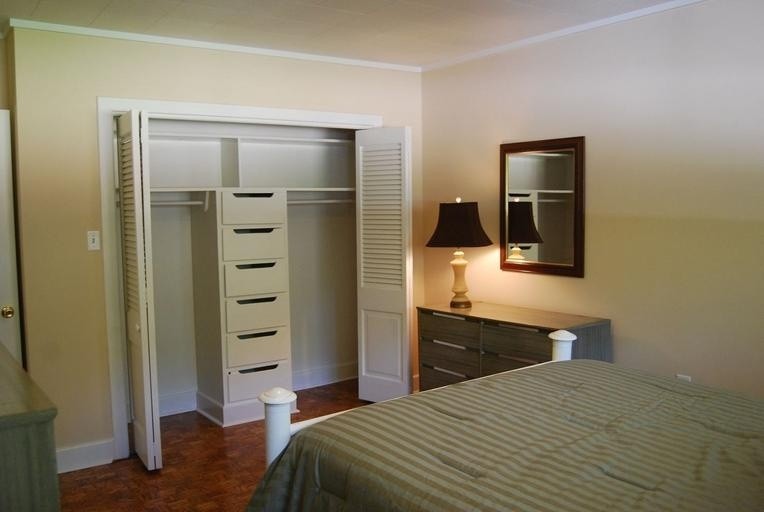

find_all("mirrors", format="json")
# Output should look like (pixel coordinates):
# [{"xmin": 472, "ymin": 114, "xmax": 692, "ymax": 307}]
[{"xmin": 498, "ymin": 136, "xmax": 586, "ymax": 279}]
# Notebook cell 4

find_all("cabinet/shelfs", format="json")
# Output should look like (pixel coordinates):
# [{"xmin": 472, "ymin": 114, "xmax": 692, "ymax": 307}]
[
  {"xmin": 418, "ymin": 307, "xmax": 611, "ymax": 392},
  {"xmin": 148, "ymin": 137, "xmax": 359, "ymax": 427},
  {"xmin": 1, "ymin": 336, "xmax": 61, "ymax": 510}
]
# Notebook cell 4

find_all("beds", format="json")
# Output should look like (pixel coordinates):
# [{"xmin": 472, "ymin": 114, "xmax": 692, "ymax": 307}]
[{"xmin": 243, "ymin": 330, "xmax": 763, "ymax": 510}]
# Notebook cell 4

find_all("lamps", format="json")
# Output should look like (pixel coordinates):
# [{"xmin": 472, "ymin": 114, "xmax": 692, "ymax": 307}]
[
  {"xmin": 509, "ymin": 197, "xmax": 544, "ymax": 262},
  {"xmin": 425, "ymin": 193, "xmax": 491, "ymax": 309}
]
